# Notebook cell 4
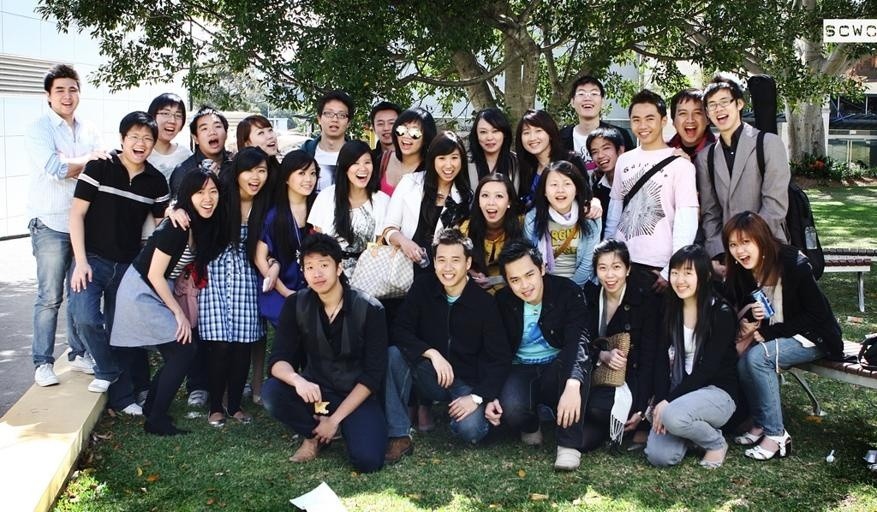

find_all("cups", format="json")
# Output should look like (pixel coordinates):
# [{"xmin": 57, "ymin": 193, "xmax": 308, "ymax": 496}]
[
  {"xmin": 750, "ymin": 289, "xmax": 774, "ymax": 319},
  {"xmin": 415, "ymin": 247, "xmax": 430, "ymax": 268}
]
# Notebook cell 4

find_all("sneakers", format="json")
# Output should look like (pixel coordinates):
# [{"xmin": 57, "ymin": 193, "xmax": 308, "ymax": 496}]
[
  {"xmin": 520, "ymin": 422, "xmax": 543, "ymax": 446},
  {"xmin": 34, "ymin": 364, "xmax": 60, "ymax": 387},
  {"xmin": 70, "ymin": 350, "xmax": 96, "ymax": 374},
  {"xmin": 554, "ymin": 444, "xmax": 582, "ymax": 471},
  {"xmin": 88, "ymin": 377, "xmax": 120, "ymax": 393},
  {"xmin": 120, "ymin": 380, "xmax": 264, "ymax": 436},
  {"xmin": 699, "ymin": 442, "xmax": 729, "ymax": 471}
]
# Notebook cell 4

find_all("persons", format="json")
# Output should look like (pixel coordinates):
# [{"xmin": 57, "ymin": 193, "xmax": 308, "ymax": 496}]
[
  {"xmin": 580, "ymin": 237, "xmax": 661, "ymax": 453},
  {"xmin": 24, "ymin": 61, "xmax": 113, "ymax": 389},
  {"xmin": 645, "ymin": 243, "xmax": 750, "ymax": 469},
  {"xmin": 483, "ymin": 237, "xmax": 595, "ymax": 473},
  {"xmin": 65, "ymin": 74, "xmax": 796, "ymax": 438},
  {"xmin": 719, "ymin": 208, "xmax": 848, "ymax": 463},
  {"xmin": 261, "ymin": 233, "xmax": 389, "ymax": 474},
  {"xmin": 382, "ymin": 228, "xmax": 514, "ymax": 465}
]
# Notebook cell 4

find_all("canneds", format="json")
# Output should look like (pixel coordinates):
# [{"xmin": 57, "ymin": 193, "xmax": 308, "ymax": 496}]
[{"xmin": 748, "ymin": 289, "xmax": 775, "ymax": 320}]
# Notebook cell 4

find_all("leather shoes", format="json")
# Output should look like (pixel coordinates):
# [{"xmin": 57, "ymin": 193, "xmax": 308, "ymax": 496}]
[
  {"xmin": 289, "ymin": 424, "xmax": 343, "ymax": 464},
  {"xmin": 627, "ymin": 439, "xmax": 646, "ymax": 453},
  {"xmin": 383, "ymin": 436, "xmax": 413, "ymax": 464},
  {"xmin": 417, "ymin": 404, "xmax": 436, "ymax": 432}
]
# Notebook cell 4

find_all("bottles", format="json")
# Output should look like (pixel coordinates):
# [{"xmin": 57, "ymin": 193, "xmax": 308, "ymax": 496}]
[{"xmin": 805, "ymin": 225, "xmax": 816, "ymax": 250}]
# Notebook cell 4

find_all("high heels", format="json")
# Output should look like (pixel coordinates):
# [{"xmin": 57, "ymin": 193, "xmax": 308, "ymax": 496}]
[{"xmin": 733, "ymin": 425, "xmax": 792, "ymax": 461}]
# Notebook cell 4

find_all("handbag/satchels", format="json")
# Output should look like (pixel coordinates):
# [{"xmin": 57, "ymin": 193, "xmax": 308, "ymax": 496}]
[
  {"xmin": 173, "ymin": 270, "xmax": 199, "ymax": 329},
  {"xmin": 734, "ymin": 304, "xmax": 759, "ymax": 358},
  {"xmin": 591, "ymin": 333, "xmax": 631, "ymax": 386},
  {"xmin": 258, "ymin": 258, "xmax": 308, "ymax": 327},
  {"xmin": 349, "ymin": 245, "xmax": 414, "ymax": 299},
  {"xmin": 857, "ymin": 333, "xmax": 877, "ymax": 371}
]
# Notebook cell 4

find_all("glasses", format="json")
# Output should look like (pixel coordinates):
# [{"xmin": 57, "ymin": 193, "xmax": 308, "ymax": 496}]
[
  {"xmin": 321, "ymin": 111, "xmax": 349, "ymax": 120},
  {"xmin": 156, "ymin": 111, "xmax": 184, "ymax": 120},
  {"xmin": 394, "ymin": 124, "xmax": 423, "ymax": 140},
  {"xmin": 574, "ymin": 89, "xmax": 601, "ymax": 98},
  {"xmin": 706, "ymin": 97, "xmax": 736, "ymax": 111},
  {"xmin": 125, "ymin": 133, "xmax": 155, "ymax": 146}
]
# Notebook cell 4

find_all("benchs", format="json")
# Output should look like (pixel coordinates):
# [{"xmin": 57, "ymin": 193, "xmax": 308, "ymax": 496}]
[
  {"xmin": 820, "ymin": 245, "xmax": 876, "ymax": 313},
  {"xmin": 788, "ymin": 334, "xmax": 876, "ymax": 418}
]
